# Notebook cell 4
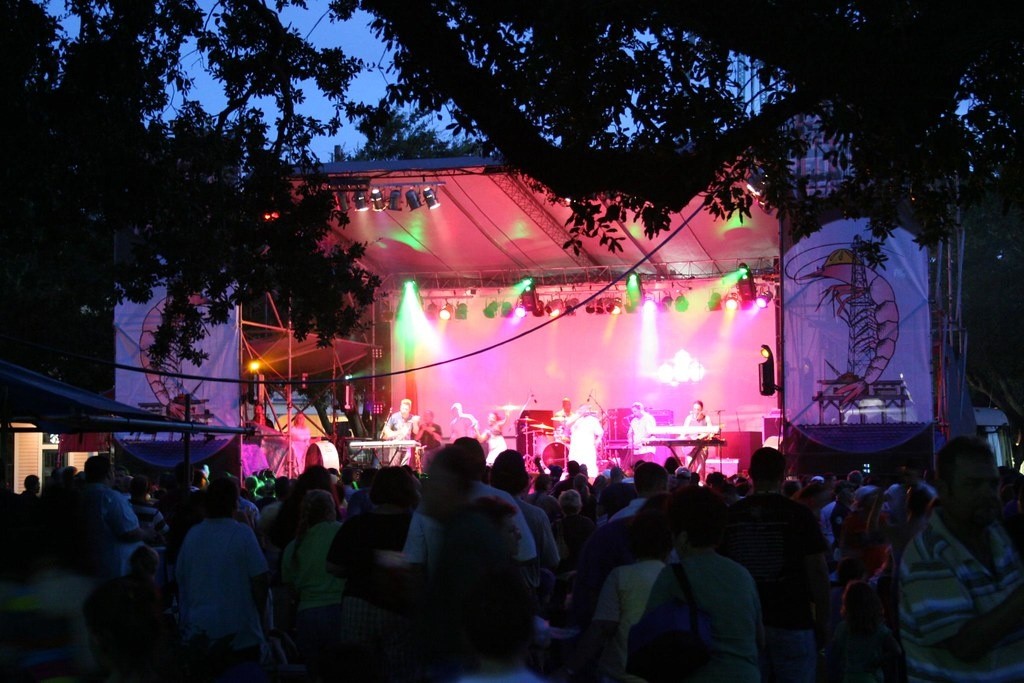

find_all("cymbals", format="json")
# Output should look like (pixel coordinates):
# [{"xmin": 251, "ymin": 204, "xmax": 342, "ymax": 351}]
[
  {"xmin": 518, "ymin": 417, "xmax": 535, "ymax": 421},
  {"xmin": 551, "ymin": 416, "xmax": 568, "ymax": 422},
  {"xmin": 530, "ymin": 422, "xmax": 554, "ymax": 430}
]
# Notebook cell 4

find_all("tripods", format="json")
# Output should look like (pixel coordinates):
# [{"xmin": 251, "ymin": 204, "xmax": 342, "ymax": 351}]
[{"xmin": 522, "ymin": 421, "xmax": 545, "ymax": 474}]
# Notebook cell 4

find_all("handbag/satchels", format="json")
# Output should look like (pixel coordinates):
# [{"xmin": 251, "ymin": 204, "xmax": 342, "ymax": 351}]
[{"xmin": 623, "ymin": 595, "xmax": 721, "ymax": 683}]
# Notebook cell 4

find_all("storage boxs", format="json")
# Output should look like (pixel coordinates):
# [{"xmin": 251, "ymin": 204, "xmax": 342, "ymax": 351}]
[{"xmin": 705, "ymin": 459, "xmax": 739, "ymax": 478}]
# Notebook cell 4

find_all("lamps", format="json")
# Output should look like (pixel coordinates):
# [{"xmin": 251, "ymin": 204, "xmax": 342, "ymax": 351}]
[
  {"xmin": 708, "ymin": 283, "xmax": 773, "ymax": 311},
  {"xmin": 483, "ymin": 289, "xmax": 688, "ymax": 318},
  {"xmin": 373, "ymin": 296, "xmax": 474, "ymax": 320},
  {"xmin": 337, "ymin": 182, "xmax": 447, "ymax": 212}
]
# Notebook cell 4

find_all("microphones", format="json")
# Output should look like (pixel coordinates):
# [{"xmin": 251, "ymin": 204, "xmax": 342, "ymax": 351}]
[
  {"xmin": 587, "ymin": 393, "xmax": 593, "ymax": 402},
  {"xmin": 390, "ymin": 408, "xmax": 392, "ymax": 417},
  {"xmin": 531, "ymin": 395, "xmax": 537, "ymax": 404},
  {"xmin": 624, "ymin": 415, "xmax": 634, "ymax": 419}
]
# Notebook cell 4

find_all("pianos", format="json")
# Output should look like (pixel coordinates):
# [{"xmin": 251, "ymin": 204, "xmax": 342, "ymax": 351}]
[
  {"xmin": 602, "ymin": 442, "xmax": 638, "ymax": 449},
  {"xmin": 640, "ymin": 425, "xmax": 726, "ymax": 446},
  {"xmin": 350, "ymin": 436, "xmax": 422, "ymax": 451}
]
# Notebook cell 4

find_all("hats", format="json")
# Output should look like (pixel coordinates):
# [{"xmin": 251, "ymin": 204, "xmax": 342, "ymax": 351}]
[
  {"xmin": 533, "ymin": 494, "xmax": 566, "ymax": 523},
  {"xmin": 854, "ymin": 485, "xmax": 879, "ymax": 501},
  {"xmin": 811, "ymin": 475, "xmax": 824, "ymax": 483},
  {"xmin": 558, "ymin": 488, "xmax": 583, "ymax": 514}
]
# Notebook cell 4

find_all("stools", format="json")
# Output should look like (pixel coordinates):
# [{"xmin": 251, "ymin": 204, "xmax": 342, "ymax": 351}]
[{"xmin": 859, "ymin": 398, "xmax": 887, "ymax": 423}]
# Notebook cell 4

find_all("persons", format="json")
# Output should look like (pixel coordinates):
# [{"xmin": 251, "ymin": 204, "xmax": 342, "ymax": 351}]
[
  {"xmin": 247, "ymin": 399, "xmax": 713, "ymax": 485},
  {"xmin": 0, "ymin": 434, "xmax": 1024, "ymax": 683}
]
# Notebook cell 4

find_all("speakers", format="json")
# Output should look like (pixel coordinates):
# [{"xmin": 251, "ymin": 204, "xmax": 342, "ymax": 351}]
[
  {"xmin": 717, "ymin": 431, "xmax": 762, "ymax": 470},
  {"xmin": 516, "ymin": 410, "xmax": 554, "ymax": 456},
  {"xmin": 248, "ymin": 374, "xmax": 264, "ymax": 404},
  {"xmin": 608, "ymin": 408, "xmax": 634, "ymax": 440},
  {"xmin": 765, "ymin": 418, "xmax": 783, "ymax": 443},
  {"xmin": 650, "ymin": 409, "xmax": 674, "ymax": 466},
  {"xmin": 759, "ymin": 362, "xmax": 775, "ymax": 396}
]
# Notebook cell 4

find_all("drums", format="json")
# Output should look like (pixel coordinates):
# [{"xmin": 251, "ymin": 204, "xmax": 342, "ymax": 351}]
[{"xmin": 542, "ymin": 441, "xmax": 569, "ymax": 470}]
